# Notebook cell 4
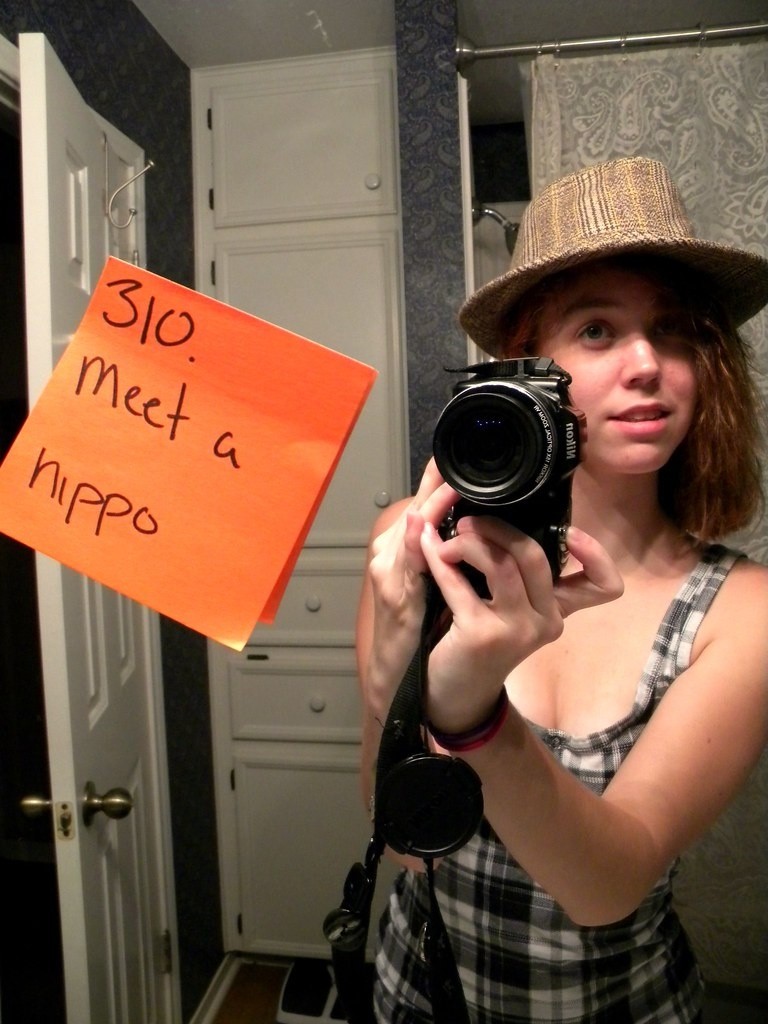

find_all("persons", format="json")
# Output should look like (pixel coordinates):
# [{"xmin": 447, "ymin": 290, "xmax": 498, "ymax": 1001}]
[{"xmin": 358, "ymin": 154, "xmax": 767, "ymax": 1024}]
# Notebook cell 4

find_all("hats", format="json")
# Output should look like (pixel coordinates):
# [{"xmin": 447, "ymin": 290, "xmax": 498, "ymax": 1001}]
[{"xmin": 460, "ymin": 157, "xmax": 768, "ymax": 363}]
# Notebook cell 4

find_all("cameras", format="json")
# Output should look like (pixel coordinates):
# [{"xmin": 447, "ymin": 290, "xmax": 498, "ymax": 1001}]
[{"xmin": 433, "ymin": 356, "xmax": 586, "ymax": 599}]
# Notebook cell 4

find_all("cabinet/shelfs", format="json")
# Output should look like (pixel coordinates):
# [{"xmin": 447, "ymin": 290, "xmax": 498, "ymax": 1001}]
[{"xmin": 192, "ymin": 43, "xmax": 413, "ymax": 961}]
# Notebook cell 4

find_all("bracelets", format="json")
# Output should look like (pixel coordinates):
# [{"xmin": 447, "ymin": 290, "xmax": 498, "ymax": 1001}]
[{"xmin": 429, "ymin": 681, "xmax": 511, "ymax": 753}]
